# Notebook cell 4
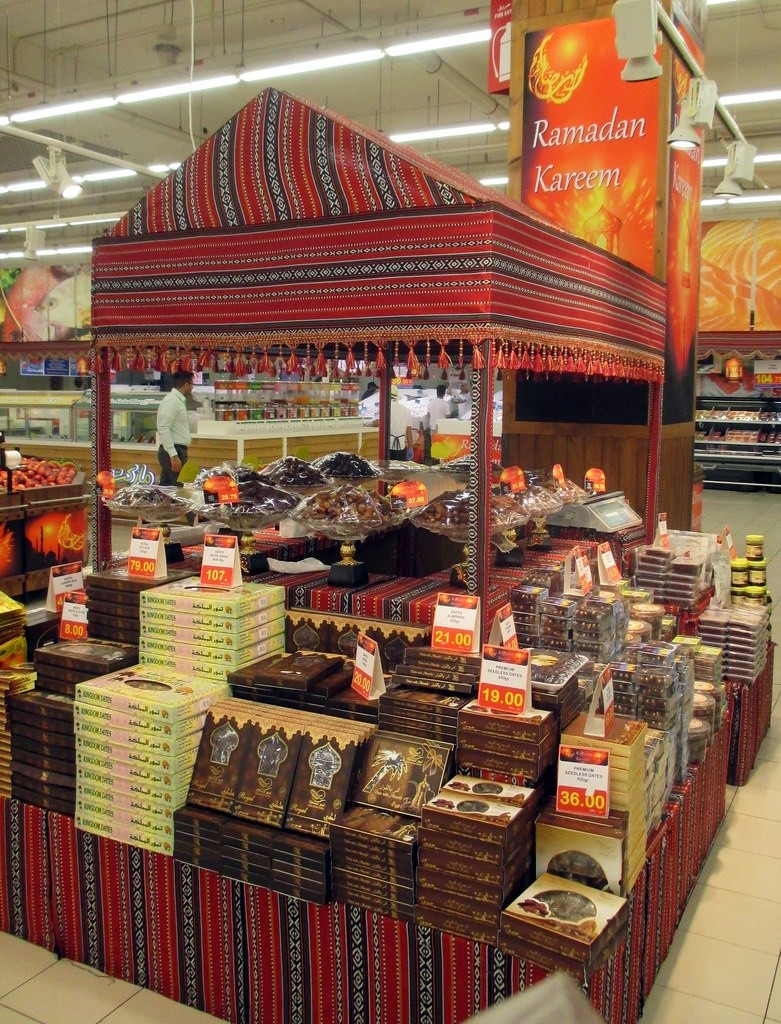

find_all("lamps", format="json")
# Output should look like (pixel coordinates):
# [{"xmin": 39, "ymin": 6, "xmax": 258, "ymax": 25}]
[
  {"xmin": 611, "ymin": 0, "xmax": 663, "ymax": 82},
  {"xmin": 23, "ymin": 225, "xmax": 46, "ymax": 261},
  {"xmin": 667, "ymin": 76, "xmax": 717, "ymax": 152},
  {"xmin": 32, "ymin": 145, "xmax": 83, "ymax": 202},
  {"xmin": 714, "ymin": 140, "xmax": 758, "ymax": 201}
]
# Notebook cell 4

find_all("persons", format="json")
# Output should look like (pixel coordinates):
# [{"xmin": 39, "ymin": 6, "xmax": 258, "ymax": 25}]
[
  {"xmin": 450, "ymin": 378, "xmax": 471, "ymax": 420},
  {"xmin": 370, "ymin": 384, "xmax": 414, "ymax": 461},
  {"xmin": 426, "ymin": 384, "xmax": 450, "ymax": 466},
  {"xmin": 362, "ymin": 382, "xmax": 380, "ymax": 400},
  {"xmin": 156, "ymin": 370, "xmax": 193, "ymax": 486}
]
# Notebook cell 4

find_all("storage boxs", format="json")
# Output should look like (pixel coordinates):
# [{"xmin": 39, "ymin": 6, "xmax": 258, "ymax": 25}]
[
  {"xmin": 0, "ymin": 560, "xmax": 649, "ymax": 984},
  {"xmin": 20, "ymin": 483, "xmax": 89, "ymax": 605},
  {"xmin": 1, "ymin": 490, "xmax": 28, "ymax": 606}
]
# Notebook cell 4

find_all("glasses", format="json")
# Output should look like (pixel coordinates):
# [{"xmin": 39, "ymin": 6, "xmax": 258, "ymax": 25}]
[{"xmin": 188, "ymin": 382, "xmax": 194, "ymax": 387}]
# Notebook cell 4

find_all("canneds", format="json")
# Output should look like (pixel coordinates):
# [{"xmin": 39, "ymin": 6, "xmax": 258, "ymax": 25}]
[{"xmin": 730, "ymin": 534, "xmax": 773, "ymax": 647}]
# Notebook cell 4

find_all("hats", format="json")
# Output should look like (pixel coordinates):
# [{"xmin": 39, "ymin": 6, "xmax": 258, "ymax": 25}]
[
  {"xmin": 367, "ymin": 382, "xmax": 379, "ymax": 388},
  {"xmin": 391, "ymin": 385, "xmax": 401, "ymax": 400}
]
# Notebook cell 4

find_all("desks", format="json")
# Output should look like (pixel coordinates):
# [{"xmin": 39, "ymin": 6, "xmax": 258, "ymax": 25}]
[{"xmin": 1, "ymin": 641, "xmax": 775, "ymax": 1024}]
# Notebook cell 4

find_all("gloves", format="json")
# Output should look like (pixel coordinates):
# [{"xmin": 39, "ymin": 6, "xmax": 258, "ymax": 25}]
[{"xmin": 407, "ymin": 448, "xmax": 413, "ymax": 460}]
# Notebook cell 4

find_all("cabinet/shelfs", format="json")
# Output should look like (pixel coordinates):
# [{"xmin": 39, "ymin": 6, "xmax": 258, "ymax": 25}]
[{"xmin": 695, "ymin": 396, "xmax": 781, "ymax": 494}]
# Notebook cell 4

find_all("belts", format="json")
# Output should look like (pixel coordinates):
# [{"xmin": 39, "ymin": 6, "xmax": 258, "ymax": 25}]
[{"xmin": 160, "ymin": 444, "xmax": 187, "ymax": 450}]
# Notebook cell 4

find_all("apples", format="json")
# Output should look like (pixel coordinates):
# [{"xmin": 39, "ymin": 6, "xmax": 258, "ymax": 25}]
[{"xmin": 0, "ymin": 457, "xmax": 76, "ymax": 491}]
